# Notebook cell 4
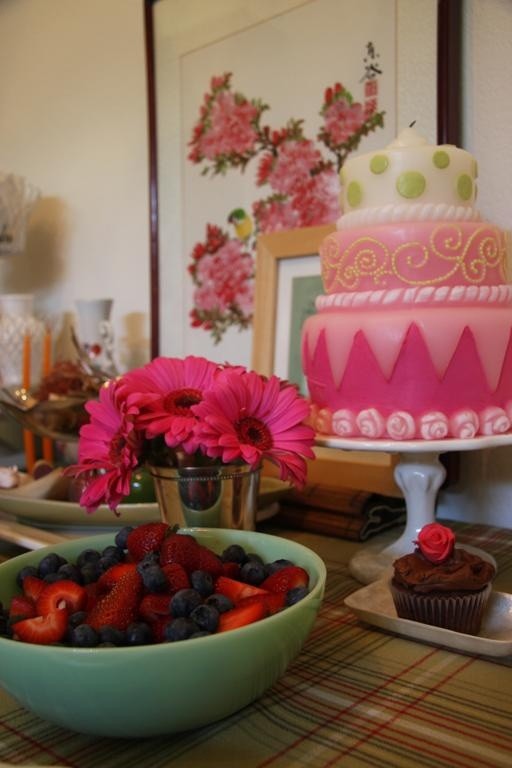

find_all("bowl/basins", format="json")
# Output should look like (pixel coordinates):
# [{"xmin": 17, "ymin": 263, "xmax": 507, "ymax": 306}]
[{"xmin": 0, "ymin": 526, "xmax": 328, "ymax": 738}]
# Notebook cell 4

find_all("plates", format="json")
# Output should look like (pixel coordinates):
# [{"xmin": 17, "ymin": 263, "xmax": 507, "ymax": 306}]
[{"xmin": 0, "ymin": 458, "xmax": 298, "ymax": 551}]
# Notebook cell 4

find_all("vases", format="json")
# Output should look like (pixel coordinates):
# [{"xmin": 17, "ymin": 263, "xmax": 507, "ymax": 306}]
[{"xmin": 143, "ymin": 448, "xmax": 261, "ymax": 531}]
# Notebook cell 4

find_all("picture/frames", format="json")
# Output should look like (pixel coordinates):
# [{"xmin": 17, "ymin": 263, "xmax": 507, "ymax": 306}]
[
  {"xmin": 146, "ymin": 1, "xmax": 465, "ymax": 376},
  {"xmin": 249, "ymin": 224, "xmax": 404, "ymax": 499}
]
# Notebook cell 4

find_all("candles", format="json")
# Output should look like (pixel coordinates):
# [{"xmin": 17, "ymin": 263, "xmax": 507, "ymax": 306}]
[{"xmin": 23, "ymin": 331, "xmax": 61, "ymax": 468}]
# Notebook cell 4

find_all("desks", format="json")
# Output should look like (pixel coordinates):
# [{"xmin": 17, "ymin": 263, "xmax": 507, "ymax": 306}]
[{"xmin": 1, "ymin": 506, "xmax": 512, "ymax": 763}]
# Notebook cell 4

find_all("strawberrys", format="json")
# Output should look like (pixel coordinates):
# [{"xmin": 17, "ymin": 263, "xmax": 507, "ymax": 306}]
[{"xmin": 8, "ymin": 524, "xmax": 309, "ymax": 645}]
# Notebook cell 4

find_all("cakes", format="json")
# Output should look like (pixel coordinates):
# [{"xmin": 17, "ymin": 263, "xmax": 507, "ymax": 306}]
[
  {"xmin": 388, "ymin": 524, "xmax": 497, "ymax": 635},
  {"xmin": 298, "ymin": 119, "xmax": 512, "ymax": 441}
]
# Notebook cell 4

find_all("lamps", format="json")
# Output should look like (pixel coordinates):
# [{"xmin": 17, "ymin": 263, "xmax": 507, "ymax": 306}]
[{"xmin": 296, "ymin": 120, "xmax": 511, "ymax": 586}]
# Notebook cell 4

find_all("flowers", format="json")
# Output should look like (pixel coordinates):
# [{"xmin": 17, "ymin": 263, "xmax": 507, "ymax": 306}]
[{"xmin": 64, "ymin": 339, "xmax": 311, "ymax": 515}]
